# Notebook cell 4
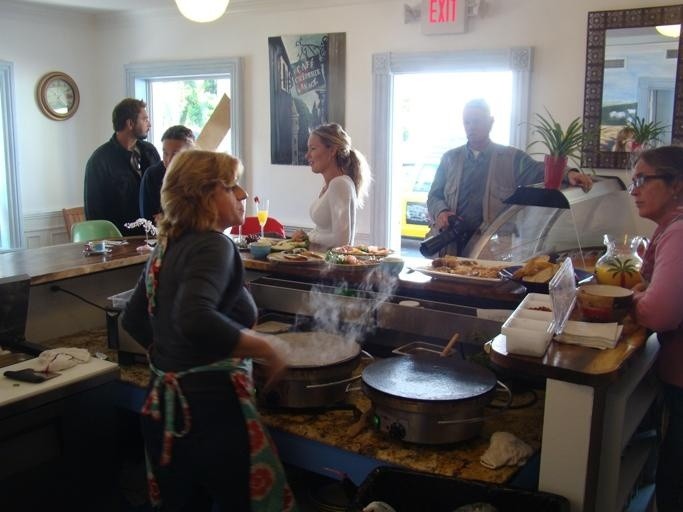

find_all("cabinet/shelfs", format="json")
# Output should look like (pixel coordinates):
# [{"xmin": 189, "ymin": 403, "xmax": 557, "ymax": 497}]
[
  {"xmin": 462, "ymin": 177, "xmax": 646, "ymax": 275},
  {"xmin": 542, "ymin": 289, "xmax": 659, "ymax": 510}
]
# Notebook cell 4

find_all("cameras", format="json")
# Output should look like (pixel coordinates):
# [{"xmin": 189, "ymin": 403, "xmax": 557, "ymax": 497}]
[{"xmin": 418, "ymin": 216, "xmax": 472, "ymax": 258}]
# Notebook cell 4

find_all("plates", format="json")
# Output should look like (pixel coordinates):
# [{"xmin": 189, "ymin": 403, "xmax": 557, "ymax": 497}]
[
  {"xmin": 332, "ymin": 245, "xmax": 389, "ymax": 257},
  {"xmin": 326, "ymin": 255, "xmax": 382, "ymax": 269},
  {"xmin": 268, "ymin": 251, "xmax": 325, "ymax": 264},
  {"xmin": 499, "ymin": 263, "xmax": 594, "ymax": 292},
  {"xmin": 258, "ymin": 238, "xmax": 309, "ymax": 251},
  {"xmin": 408, "ymin": 259, "xmax": 525, "ymax": 284}
]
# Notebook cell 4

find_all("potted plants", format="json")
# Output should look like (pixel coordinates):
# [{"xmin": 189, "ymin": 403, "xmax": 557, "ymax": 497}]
[
  {"xmin": 519, "ymin": 110, "xmax": 611, "ymax": 189},
  {"xmin": 617, "ymin": 108, "xmax": 666, "ymax": 150}
]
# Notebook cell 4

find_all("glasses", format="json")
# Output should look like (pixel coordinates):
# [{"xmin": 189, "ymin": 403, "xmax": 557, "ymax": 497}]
[{"xmin": 627, "ymin": 174, "xmax": 674, "ymax": 189}]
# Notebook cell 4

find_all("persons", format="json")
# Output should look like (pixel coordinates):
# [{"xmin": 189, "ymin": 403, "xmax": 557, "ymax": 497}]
[
  {"xmin": 140, "ymin": 125, "xmax": 194, "ymax": 234},
  {"xmin": 292, "ymin": 123, "xmax": 367, "ymax": 250},
  {"xmin": 83, "ymin": 98, "xmax": 159, "ymax": 237},
  {"xmin": 121, "ymin": 149, "xmax": 295, "ymax": 512},
  {"xmin": 629, "ymin": 146, "xmax": 683, "ymax": 512},
  {"xmin": 613, "ymin": 127, "xmax": 638, "ymax": 153},
  {"xmin": 423, "ymin": 98, "xmax": 592, "ymax": 259}
]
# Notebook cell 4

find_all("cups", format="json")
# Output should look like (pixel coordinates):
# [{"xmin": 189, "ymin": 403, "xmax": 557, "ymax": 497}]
[
  {"xmin": 234, "ymin": 199, "xmax": 247, "ymax": 243},
  {"xmin": 256, "ymin": 199, "xmax": 270, "ymax": 238},
  {"xmin": 89, "ymin": 241, "xmax": 106, "ymax": 253}
]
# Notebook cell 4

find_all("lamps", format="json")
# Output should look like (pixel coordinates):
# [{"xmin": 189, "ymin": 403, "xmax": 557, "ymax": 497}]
[{"xmin": 175, "ymin": 0, "xmax": 229, "ymax": 23}]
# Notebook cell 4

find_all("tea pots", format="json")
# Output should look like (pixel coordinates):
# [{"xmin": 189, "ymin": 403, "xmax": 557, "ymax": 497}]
[{"xmin": 596, "ymin": 233, "xmax": 653, "ymax": 290}]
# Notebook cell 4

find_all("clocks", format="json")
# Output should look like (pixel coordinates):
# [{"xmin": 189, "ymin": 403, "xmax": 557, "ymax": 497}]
[{"xmin": 36, "ymin": 72, "xmax": 80, "ymax": 120}]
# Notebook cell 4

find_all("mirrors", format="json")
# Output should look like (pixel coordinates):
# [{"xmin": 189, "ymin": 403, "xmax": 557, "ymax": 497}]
[{"xmin": 579, "ymin": 5, "xmax": 683, "ymax": 170}]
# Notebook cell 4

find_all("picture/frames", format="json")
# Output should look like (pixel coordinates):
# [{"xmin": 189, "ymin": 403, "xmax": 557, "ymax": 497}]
[{"xmin": 267, "ymin": 33, "xmax": 344, "ymax": 166}]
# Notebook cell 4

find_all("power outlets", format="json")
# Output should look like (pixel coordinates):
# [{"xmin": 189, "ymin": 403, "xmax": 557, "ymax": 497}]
[{"xmin": 48, "ymin": 284, "xmax": 61, "ymax": 308}]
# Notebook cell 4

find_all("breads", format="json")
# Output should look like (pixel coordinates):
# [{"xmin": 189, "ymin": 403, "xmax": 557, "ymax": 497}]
[{"xmin": 511, "ymin": 255, "xmax": 559, "ymax": 283}]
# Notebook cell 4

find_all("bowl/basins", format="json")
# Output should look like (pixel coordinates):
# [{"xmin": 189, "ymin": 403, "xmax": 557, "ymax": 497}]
[
  {"xmin": 580, "ymin": 306, "xmax": 630, "ymax": 324},
  {"xmin": 250, "ymin": 242, "xmax": 271, "ymax": 258},
  {"xmin": 382, "ymin": 260, "xmax": 405, "ymax": 276},
  {"xmin": 578, "ymin": 284, "xmax": 634, "ymax": 307}
]
